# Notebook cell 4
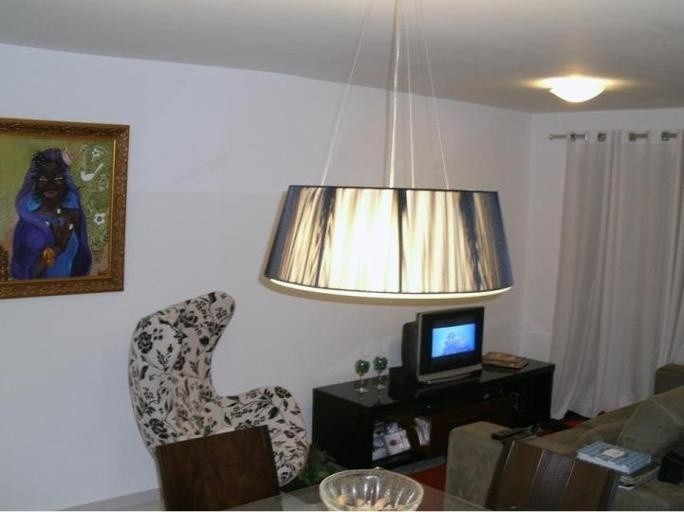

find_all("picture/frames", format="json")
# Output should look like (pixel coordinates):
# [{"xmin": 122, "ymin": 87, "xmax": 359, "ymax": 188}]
[{"xmin": 0, "ymin": 117, "xmax": 131, "ymax": 300}]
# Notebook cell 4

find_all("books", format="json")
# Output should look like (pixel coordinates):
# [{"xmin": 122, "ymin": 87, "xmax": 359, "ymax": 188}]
[{"xmin": 574, "ymin": 436, "xmax": 662, "ymax": 491}]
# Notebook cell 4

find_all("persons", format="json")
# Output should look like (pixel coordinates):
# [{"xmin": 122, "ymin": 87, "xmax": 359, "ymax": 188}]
[
  {"xmin": 442, "ymin": 329, "xmax": 470, "ymax": 355},
  {"xmin": 9, "ymin": 145, "xmax": 93, "ymax": 279}
]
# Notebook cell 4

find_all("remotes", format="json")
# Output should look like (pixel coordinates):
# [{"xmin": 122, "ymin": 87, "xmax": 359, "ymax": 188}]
[{"xmin": 490, "ymin": 424, "xmax": 524, "ymax": 440}]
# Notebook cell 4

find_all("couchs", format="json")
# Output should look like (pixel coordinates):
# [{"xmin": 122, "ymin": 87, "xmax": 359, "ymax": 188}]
[{"xmin": 443, "ymin": 360, "xmax": 683, "ymax": 510}]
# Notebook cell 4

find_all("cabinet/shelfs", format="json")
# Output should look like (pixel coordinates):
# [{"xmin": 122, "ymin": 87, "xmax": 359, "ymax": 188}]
[{"xmin": 311, "ymin": 348, "xmax": 555, "ymax": 471}]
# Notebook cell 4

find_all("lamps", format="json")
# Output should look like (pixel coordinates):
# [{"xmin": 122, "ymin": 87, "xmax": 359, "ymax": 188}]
[
  {"xmin": 548, "ymin": 75, "xmax": 607, "ymax": 105},
  {"xmin": 258, "ymin": 1, "xmax": 516, "ymax": 303}
]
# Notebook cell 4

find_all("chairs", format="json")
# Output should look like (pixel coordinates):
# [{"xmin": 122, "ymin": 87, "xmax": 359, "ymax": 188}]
[
  {"xmin": 485, "ymin": 436, "xmax": 619, "ymax": 511},
  {"xmin": 152, "ymin": 424, "xmax": 280, "ymax": 511},
  {"xmin": 125, "ymin": 289, "xmax": 308, "ymax": 492}
]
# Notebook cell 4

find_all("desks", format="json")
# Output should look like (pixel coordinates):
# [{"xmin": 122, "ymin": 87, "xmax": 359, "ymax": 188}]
[{"xmin": 220, "ymin": 466, "xmax": 489, "ymax": 510}]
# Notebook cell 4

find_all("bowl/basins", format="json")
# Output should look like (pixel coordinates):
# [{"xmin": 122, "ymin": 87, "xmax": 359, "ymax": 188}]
[{"xmin": 317, "ymin": 468, "xmax": 423, "ymax": 511}]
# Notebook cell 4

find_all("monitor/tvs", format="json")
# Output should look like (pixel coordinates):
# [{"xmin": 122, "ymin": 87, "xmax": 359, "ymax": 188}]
[{"xmin": 400, "ymin": 304, "xmax": 485, "ymax": 385}]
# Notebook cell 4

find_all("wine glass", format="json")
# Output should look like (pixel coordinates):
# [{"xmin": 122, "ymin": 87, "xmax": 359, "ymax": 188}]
[
  {"xmin": 353, "ymin": 360, "xmax": 370, "ymax": 393},
  {"xmin": 372, "ymin": 356, "xmax": 388, "ymax": 390}
]
